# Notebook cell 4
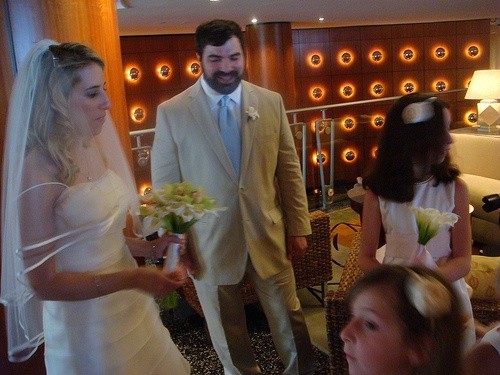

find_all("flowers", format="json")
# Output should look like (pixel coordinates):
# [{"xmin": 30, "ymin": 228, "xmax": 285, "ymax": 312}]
[
  {"xmin": 412, "ymin": 206, "xmax": 461, "ymax": 270},
  {"xmin": 245, "ymin": 106, "xmax": 260, "ymax": 122},
  {"xmin": 135, "ymin": 179, "xmax": 229, "ymax": 311}
]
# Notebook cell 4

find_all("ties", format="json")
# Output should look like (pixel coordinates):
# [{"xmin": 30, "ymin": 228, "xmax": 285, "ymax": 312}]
[{"xmin": 219, "ymin": 95, "xmax": 241, "ymax": 172}]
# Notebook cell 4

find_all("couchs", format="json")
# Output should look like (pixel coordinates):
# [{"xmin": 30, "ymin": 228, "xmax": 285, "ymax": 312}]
[{"xmin": 447, "ymin": 133, "xmax": 500, "ymax": 248}]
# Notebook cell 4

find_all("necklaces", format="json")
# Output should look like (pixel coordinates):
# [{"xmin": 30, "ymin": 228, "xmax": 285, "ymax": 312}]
[{"xmin": 78, "ymin": 164, "xmax": 92, "ymax": 181}]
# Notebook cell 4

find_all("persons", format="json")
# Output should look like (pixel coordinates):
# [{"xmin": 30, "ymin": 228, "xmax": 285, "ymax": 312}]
[
  {"xmin": 149, "ymin": 19, "xmax": 317, "ymax": 375},
  {"xmin": 0, "ymin": 38, "xmax": 188, "ymax": 375},
  {"xmin": 339, "ymin": 93, "xmax": 500, "ymax": 375}
]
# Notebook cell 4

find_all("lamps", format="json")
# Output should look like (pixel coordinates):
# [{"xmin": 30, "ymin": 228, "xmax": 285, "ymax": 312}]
[{"xmin": 463, "ymin": 69, "xmax": 500, "ymax": 135}]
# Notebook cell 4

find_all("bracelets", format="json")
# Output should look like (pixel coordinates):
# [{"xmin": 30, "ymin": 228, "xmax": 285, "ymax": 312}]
[
  {"xmin": 150, "ymin": 239, "xmax": 159, "ymax": 258},
  {"xmin": 94, "ymin": 274, "xmax": 105, "ymax": 296}
]
